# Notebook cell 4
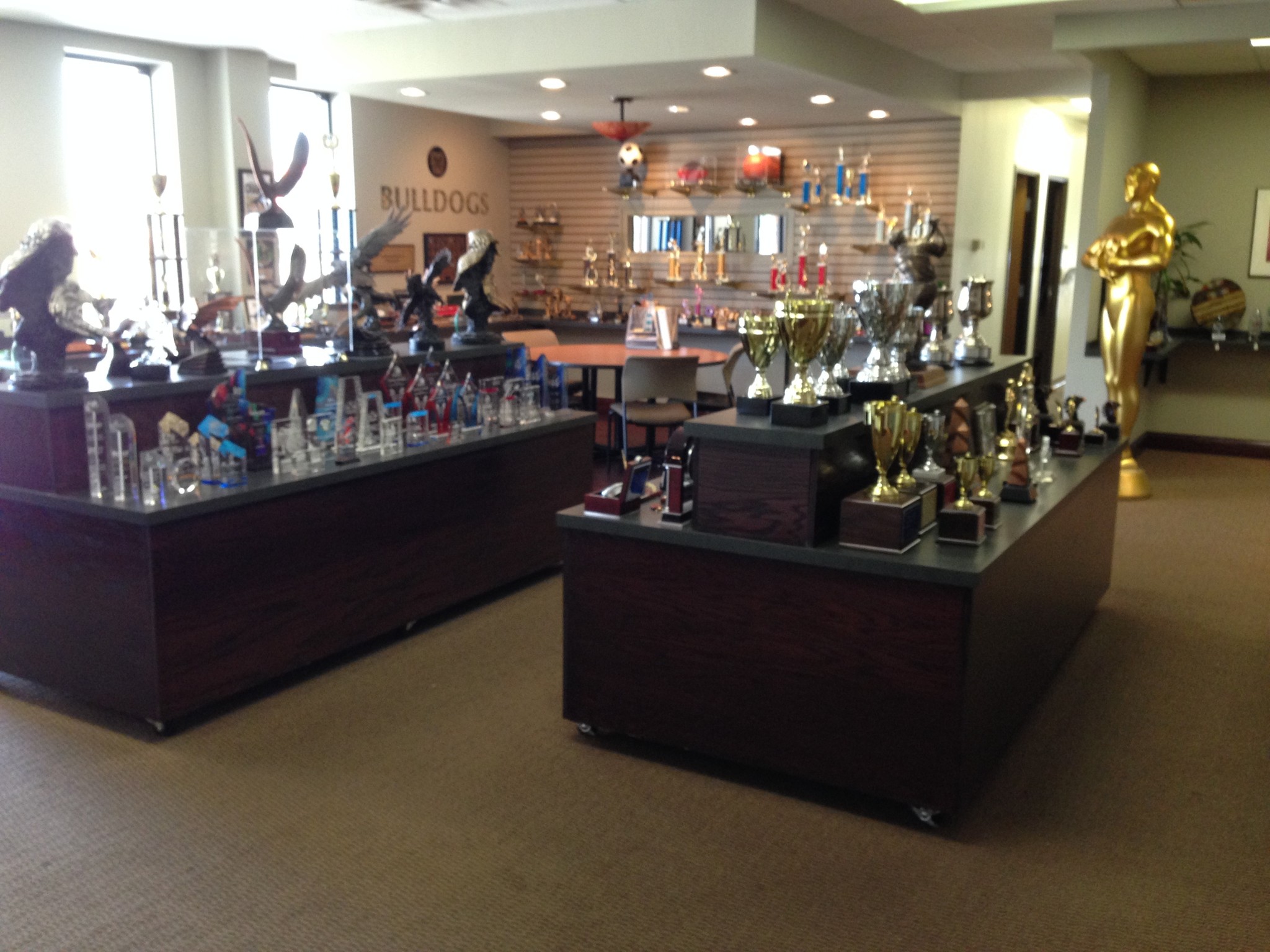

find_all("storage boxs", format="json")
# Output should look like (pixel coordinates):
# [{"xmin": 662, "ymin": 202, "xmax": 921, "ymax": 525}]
[
  {"xmin": 662, "ymin": 462, "xmax": 695, "ymax": 523},
  {"xmin": 583, "ymin": 457, "xmax": 651, "ymax": 519}
]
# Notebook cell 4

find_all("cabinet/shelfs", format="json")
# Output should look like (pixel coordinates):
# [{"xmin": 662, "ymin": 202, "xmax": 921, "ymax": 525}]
[
  {"xmin": 556, "ymin": 356, "xmax": 1129, "ymax": 833},
  {"xmin": 1, "ymin": 340, "xmax": 596, "ymax": 744},
  {"xmin": 569, "ymin": 180, "xmax": 895, "ymax": 300},
  {"xmin": 513, "ymin": 222, "xmax": 564, "ymax": 303}
]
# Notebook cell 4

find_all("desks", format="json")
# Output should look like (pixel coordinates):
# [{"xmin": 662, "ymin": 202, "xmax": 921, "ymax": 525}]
[{"xmin": 528, "ymin": 344, "xmax": 727, "ymax": 447}]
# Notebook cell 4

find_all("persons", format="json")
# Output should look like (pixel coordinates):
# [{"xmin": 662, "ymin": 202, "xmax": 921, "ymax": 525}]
[{"xmin": 1081, "ymin": 162, "xmax": 1174, "ymax": 469}]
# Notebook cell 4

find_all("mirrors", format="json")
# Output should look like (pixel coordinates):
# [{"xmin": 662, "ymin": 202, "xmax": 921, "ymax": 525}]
[{"xmin": 629, "ymin": 211, "xmax": 784, "ymax": 255}]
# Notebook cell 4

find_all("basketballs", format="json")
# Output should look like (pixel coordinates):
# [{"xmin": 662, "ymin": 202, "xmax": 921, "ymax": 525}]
[{"xmin": 743, "ymin": 152, "xmax": 780, "ymax": 181}]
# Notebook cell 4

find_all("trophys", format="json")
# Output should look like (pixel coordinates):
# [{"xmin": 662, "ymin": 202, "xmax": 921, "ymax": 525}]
[
  {"xmin": 735, "ymin": 188, "xmax": 995, "ymax": 429},
  {"xmin": 84, "ymin": 348, "xmax": 567, "ymax": 507},
  {"xmin": 839, "ymin": 361, "xmax": 1120, "ymax": 554},
  {"xmin": 512, "ymin": 148, "xmax": 873, "ymax": 330}
]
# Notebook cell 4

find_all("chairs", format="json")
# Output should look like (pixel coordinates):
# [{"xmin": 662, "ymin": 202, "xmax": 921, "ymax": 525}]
[
  {"xmin": 608, "ymin": 355, "xmax": 700, "ymax": 474},
  {"xmin": 502, "ymin": 329, "xmax": 582, "ymax": 398},
  {"xmin": 668, "ymin": 342, "xmax": 744, "ymax": 437}
]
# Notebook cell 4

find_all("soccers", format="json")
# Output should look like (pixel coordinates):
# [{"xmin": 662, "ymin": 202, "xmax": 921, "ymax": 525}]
[{"xmin": 618, "ymin": 143, "xmax": 645, "ymax": 169}]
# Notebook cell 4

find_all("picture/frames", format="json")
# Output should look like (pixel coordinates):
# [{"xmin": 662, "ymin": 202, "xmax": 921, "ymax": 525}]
[
  {"xmin": 241, "ymin": 236, "xmax": 278, "ymax": 327},
  {"xmin": 1247, "ymin": 189, "xmax": 1270, "ymax": 278},
  {"xmin": 240, "ymin": 168, "xmax": 275, "ymax": 229},
  {"xmin": 424, "ymin": 232, "xmax": 468, "ymax": 284}
]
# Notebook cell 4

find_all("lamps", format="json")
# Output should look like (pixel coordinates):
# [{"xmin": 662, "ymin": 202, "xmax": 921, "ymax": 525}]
[{"xmin": 590, "ymin": 95, "xmax": 651, "ymax": 142}]
